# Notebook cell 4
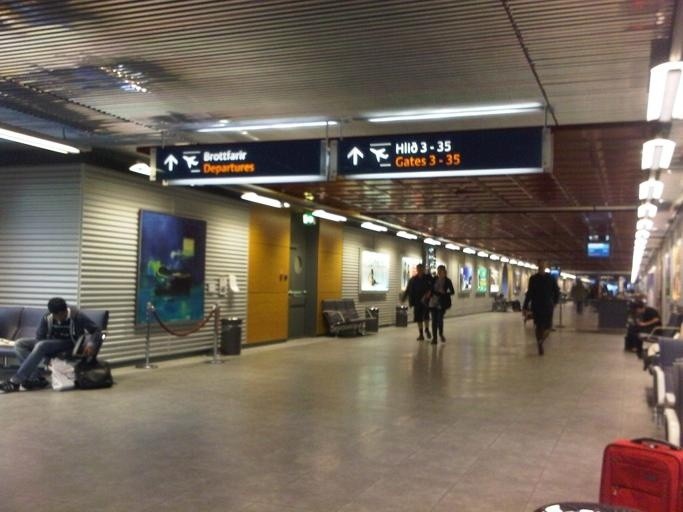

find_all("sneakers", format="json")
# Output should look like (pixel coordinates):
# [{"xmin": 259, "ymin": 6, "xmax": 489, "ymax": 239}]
[{"xmin": 0, "ymin": 378, "xmax": 48, "ymax": 392}]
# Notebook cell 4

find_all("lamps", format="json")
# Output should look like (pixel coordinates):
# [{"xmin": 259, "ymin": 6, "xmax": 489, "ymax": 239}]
[{"xmin": 635, "ymin": 1, "xmax": 682, "ymax": 241}]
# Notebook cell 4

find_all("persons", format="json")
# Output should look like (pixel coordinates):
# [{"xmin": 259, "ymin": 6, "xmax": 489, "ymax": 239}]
[
  {"xmin": 403, "ymin": 264, "xmax": 455, "ymax": 345},
  {"xmin": 628, "ymin": 299, "xmax": 662, "ymax": 353},
  {"xmin": 0, "ymin": 298, "xmax": 102, "ymax": 391},
  {"xmin": 522, "ymin": 256, "xmax": 560, "ymax": 356}
]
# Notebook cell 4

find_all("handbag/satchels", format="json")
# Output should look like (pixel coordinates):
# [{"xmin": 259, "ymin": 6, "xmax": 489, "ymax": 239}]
[
  {"xmin": 49, "ymin": 359, "xmax": 112, "ymax": 391},
  {"xmin": 421, "ymin": 295, "xmax": 430, "ymax": 305},
  {"xmin": 440, "ymin": 294, "xmax": 451, "ymax": 309}
]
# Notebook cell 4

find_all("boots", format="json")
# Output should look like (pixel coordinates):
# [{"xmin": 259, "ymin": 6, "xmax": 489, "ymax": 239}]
[{"xmin": 416, "ymin": 328, "xmax": 445, "ymax": 345}]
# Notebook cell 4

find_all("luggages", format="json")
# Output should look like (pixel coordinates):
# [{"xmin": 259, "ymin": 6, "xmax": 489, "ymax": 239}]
[{"xmin": 599, "ymin": 437, "xmax": 683, "ymax": 512}]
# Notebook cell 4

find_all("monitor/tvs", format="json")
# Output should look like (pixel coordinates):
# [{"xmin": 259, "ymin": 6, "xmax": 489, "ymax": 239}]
[{"xmin": 586, "ymin": 241, "xmax": 612, "ymax": 258}]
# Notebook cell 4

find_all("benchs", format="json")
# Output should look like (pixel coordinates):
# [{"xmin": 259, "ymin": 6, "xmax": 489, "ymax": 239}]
[
  {"xmin": 323, "ymin": 298, "xmax": 376, "ymax": 337},
  {"xmin": 492, "ymin": 300, "xmax": 518, "ymax": 312}
]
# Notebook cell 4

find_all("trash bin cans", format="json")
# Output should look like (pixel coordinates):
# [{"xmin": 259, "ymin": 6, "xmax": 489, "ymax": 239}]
[
  {"xmin": 366, "ymin": 306, "xmax": 379, "ymax": 332},
  {"xmin": 220, "ymin": 318, "xmax": 241, "ymax": 355},
  {"xmin": 396, "ymin": 305, "xmax": 408, "ymax": 327}
]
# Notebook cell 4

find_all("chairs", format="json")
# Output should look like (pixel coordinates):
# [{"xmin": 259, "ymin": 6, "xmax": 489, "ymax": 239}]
[
  {"xmin": 634, "ymin": 312, "xmax": 682, "ymax": 450},
  {"xmin": 0, "ymin": 307, "xmax": 112, "ymax": 390}
]
